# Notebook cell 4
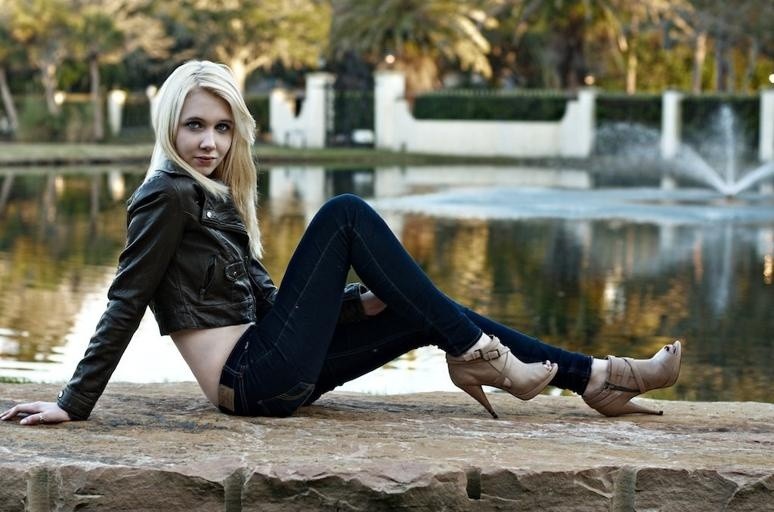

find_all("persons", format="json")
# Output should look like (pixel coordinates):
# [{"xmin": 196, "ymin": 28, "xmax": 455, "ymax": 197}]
[{"xmin": 0, "ymin": 57, "xmax": 686, "ymax": 430}]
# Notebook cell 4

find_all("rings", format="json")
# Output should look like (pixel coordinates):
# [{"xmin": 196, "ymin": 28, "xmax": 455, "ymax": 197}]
[{"xmin": 38, "ymin": 414, "xmax": 44, "ymax": 423}]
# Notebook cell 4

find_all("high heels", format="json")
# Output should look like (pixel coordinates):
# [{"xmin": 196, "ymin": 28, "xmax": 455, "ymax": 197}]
[
  {"xmin": 446, "ymin": 335, "xmax": 559, "ymax": 419},
  {"xmin": 582, "ymin": 340, "xmax": 682, "ymax": 417}
]
